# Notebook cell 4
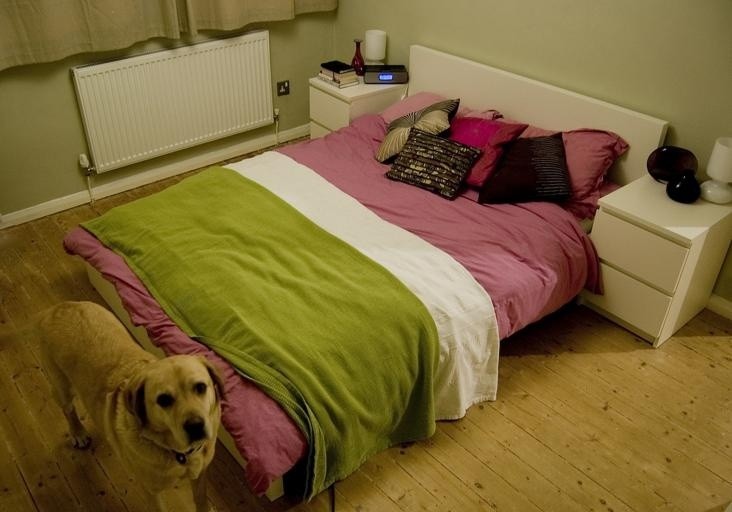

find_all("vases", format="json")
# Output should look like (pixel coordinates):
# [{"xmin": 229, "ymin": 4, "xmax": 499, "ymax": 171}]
[{"xmin": 350, "ymin": 37, "xmax": 365, "ymax": 69}]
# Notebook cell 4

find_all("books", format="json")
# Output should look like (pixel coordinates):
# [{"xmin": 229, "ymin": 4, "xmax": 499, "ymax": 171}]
[{"xmin": 319, "ymin": 60, "xmax": 360, "ymax": 89}]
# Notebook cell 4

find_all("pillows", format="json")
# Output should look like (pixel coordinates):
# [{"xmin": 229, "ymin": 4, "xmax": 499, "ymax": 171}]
[{"xmin": 373, "ymin": 92, "xmax": 631, "ymax": 220}]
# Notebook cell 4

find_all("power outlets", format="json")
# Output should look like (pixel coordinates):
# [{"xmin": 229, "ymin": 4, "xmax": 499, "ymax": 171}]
[{"xmin": 276, "ymin": 79, "xmax": 290, "ymax": 96}]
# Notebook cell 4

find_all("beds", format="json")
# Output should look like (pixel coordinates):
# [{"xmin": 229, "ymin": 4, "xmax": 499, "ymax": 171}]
[{"xmin": 62, "ymin": 38, "xmax": 673, "ymax": 511}]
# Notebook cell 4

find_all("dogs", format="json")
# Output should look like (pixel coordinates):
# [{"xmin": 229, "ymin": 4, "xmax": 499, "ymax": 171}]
[{"xmin": 33, "ymin": 298, "xmax": 227, "ymax": 512}]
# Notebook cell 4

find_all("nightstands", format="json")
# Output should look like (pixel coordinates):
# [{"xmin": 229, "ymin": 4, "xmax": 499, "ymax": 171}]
[
  {"xmin": 573, "ymin": 171, "xmax": 732, "ymax": 350},
  {"xmin": 307, "ymin": 63, "xmax": 408, "ymax": 145}
]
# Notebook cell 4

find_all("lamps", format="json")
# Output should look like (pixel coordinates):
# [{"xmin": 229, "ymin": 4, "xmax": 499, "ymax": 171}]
[{"xmin": 697, "ymin": 134, "xmax": 732, "ymax": 205}]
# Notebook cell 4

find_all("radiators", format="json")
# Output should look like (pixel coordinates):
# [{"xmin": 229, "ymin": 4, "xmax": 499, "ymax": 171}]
[{"xmin": 61, "ymin": 27, "xmax": 276, "ymax": 177}]
